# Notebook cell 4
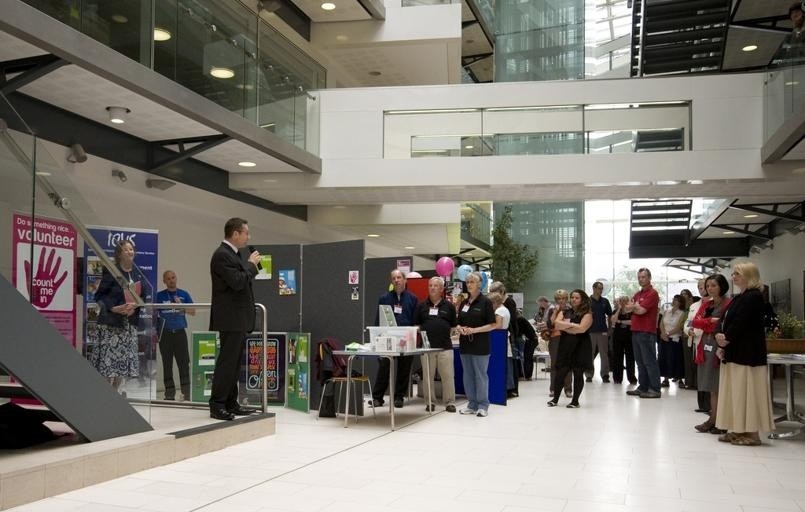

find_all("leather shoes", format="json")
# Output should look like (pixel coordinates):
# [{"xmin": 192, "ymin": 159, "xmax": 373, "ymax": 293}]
[
  {"xmin": 228, "ymin": 406, "xmax": 256, "ymax": 415},
  {"xmin": 208, "ymin": 407, "xmax": 236, "ymax": 420}
]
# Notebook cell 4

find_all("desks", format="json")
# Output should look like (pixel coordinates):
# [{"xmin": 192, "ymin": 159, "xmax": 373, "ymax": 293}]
[
  {"xmin": 330, "ymin": 347, "xmax": 445, "ymax": 433},
  {"xmin": 533, "ymin": 349, "xmax": 552, "ymax": 381},
  {"xmin": 765, "ymin": 337, "xmax": 805, "ymax": 354},
  {"xmin": 765, "ymin": 353, "xmax": 805, "ymax": 443}
]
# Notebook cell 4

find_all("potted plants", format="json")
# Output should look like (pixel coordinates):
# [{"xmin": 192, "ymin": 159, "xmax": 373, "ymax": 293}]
[{"xmin": 776, "ymin": 311, "xmax": 803, "ymax": 339}]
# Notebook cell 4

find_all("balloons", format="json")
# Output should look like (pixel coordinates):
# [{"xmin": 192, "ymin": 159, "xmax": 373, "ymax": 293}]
[
  {"xmin": 434, "ymin": 255, "xmax": 454, "ymax": 278},
  {"xmin": 457, "ymin": 264, "xmax": 473, "ymax": 283},
  {"xmin": 477, "ymin": 270, "xmax": 488, "ymax": 292}
]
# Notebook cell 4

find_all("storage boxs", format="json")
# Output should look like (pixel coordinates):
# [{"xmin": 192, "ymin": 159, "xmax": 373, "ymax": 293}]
[{"xmin": 364, "ymin": 324, "xmax": 421, "ymax": 353}]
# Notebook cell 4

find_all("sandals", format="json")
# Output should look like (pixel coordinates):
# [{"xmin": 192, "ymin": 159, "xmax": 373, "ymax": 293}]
[
  {"xmin": 678, "ymin": 380, "xmax": 685, "ymax": 388},
  {"xmin": 662, "ymin": 380, "xmax": 670, "ymax": 388}
]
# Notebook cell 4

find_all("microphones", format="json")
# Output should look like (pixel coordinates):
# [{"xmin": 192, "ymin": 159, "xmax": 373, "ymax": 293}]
[{"xmin": 249, "ymin": 246, "xmax": 263, "ymax": 271}]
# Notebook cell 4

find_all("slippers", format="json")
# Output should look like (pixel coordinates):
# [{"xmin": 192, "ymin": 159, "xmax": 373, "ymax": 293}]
[
  {"xmin": 547, "ymin": 401, "xmax": 560, "ymax": 406},
  {"xmin": 565, "ymin": 403, "xmax": 580, "ymax": 408}
]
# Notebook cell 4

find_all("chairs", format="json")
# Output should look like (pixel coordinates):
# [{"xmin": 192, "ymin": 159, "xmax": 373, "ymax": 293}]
[{"xmin": 314, "ymin": 341, "xmax": 376, "ymax": 426}]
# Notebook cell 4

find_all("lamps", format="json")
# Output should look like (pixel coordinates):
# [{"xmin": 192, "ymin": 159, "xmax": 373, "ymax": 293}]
[
  {"xmin": 107, "ymin": 106, "xmax": 128, "ymax": 125},
  {"xmin": 760, "ymin": 241, "xmax": 774, "ymax": 251},
  {"xmin": 749, "ymin": 246, "xmax": 762, "ymax": 255},
  {"xmin": 111, "ymin": 168, "xmax": 127, "ymax": 184},
  {"xmin": 168, "ymin": 0, "xmax": 318, "ymax": 103},
  {"xmin": 782, "ymin": 226, "xmax": 804, "ymax": 236},
  {"xmin": 65, "ymin": 142, "xmax": 89, "ymax": 166},
  {"xmin": 144, "ymin": 177, "xmax": 177, "ymax": 192}
]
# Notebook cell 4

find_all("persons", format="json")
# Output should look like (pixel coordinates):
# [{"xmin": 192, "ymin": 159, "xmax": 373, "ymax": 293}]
[
  {"xmin": 367, "ymin": 269, "xmax": 418, "ymax": 408},
  {"xmin": 89, "ymin": 239, "xmax": 148, "ymax": 399},
  {"xmin": 456, "ymin": 270, "xmax": 498, "ymax": 417},
  {"xmin": 487, "ymin": 280, "xmax": 520, "ymax": 399},
  {"xmin": 610, "ymin": 268, "xmax": 662, "ymax": 398},
  {"xmin": 789, "ymin": 1, "xmax": 804, "ymax": 43},
  {"xmin": 24, "ymin": 246, "xmax": 69, "ymax": 309},
  {"xmin": 484, "ymin": 291, "xmax": 511, "ymax": 407},
  {"xmin": 155, "ymin": 269, "xmax": 196, "ymax": 402},
  {"xmin": 411, "ymin": 275, "xmax": 459, "ymax": 413},
  {"xmin": 207, "ymin": 215, "xmax": 262, "ymax": 420},
  {"xmin": 659, "ymin": 263, "xmax": 777, "ymax": 446},
  {"xmin": 534, "ymin": 280, "xmax": 612, "ymax": 408}
]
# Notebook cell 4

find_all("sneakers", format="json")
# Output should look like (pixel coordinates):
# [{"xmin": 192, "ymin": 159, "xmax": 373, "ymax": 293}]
[
  {"xmin": 693, "ymin": 407, "xmax": 762, "ymax": 447},
  {"xmin": 546, "ymin": 372, "xmax": 637, "ymax": 398},
  {"xmin": 626, "ymin": 388, "xmax": 644, "ymax": 395},
  {"xmin": 638, "ymin": 388, "xmax": 662, "ymax": 398},
  {"xmin": 475, "ymin": 408, "xmax": 490, "ymax": 416},
  {"xmin": 393, "ymin": 398, "xmax": 404, "ymax": 408},
  {"xmin": 446, "ymin": 405, "xmax": 456, "ymax": 412},
  {"xmin": 459, "ymin": 407, "xmax": 477, "ymax": 416},
  {"xmin": 425, "ymin": 404, "xmax": 435, "ymax": 411},
  {"xmin": 367, "ymin": 397, "xmax": 385, "ymax": 407}
]
